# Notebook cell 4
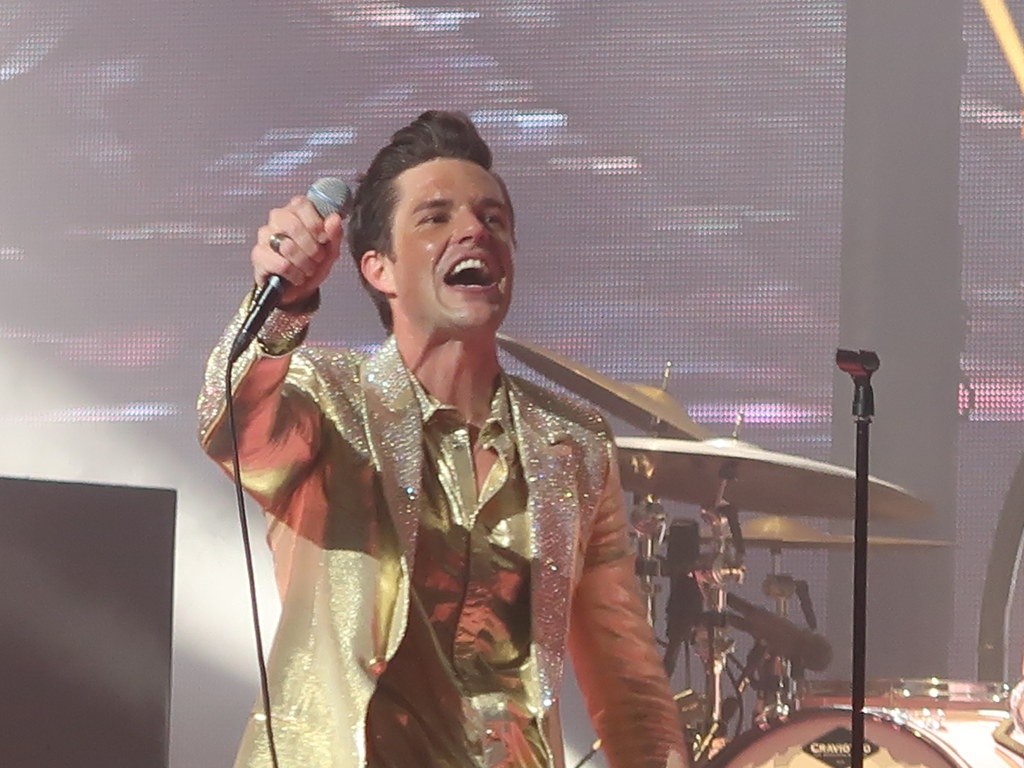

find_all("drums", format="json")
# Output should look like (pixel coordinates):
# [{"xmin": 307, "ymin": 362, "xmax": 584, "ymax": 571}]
[
  {"xmin": 891, "ymin": 677, "xmax": 1011, "ymax": 700},
  {"xmin": 704, "ymin": 704, "xmax": 1023, "ymax": 768}
]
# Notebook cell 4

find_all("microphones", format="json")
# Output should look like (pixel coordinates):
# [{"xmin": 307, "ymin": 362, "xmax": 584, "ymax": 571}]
[{"xmin": 228, "ymin": 176, "xmax": 353, "ymax": 363}]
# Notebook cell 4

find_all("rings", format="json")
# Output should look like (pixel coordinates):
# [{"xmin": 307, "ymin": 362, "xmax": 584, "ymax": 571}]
[{"xmin": 268, "ymin": 233, "xmax": 288, "ymax": 253}]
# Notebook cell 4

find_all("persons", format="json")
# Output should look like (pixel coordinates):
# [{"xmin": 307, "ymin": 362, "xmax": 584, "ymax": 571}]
[{"xmin": 195, "ymin": 111, "xmax": 694, "ymax": 768}]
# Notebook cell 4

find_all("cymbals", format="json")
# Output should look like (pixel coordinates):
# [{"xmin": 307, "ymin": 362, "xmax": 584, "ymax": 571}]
[
  {"xmin": 493, "ymin": 333, "xmax": 715, "ymax": 442},
  {"xmin": 613, "ymin": 436, "xmax": 933, "ymax": 520},
  {"xmin": 663, "ymin": 514, "xmax": 958, "ymax": 550}
]
